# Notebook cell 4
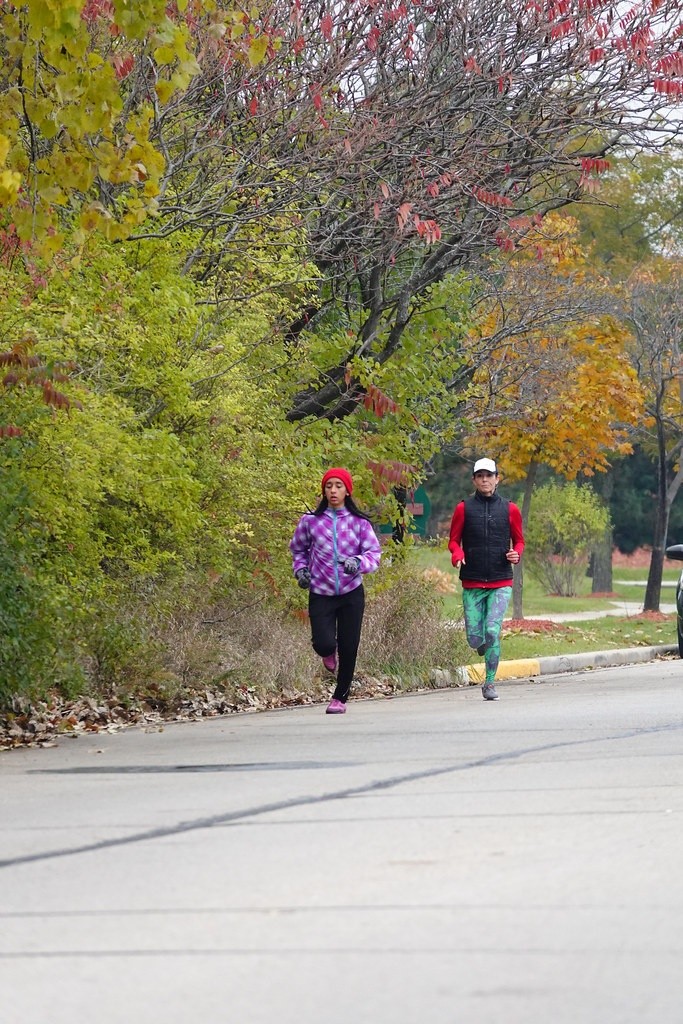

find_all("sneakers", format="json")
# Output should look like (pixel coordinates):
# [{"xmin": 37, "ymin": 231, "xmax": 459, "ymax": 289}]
[
  {"xmin": 322, "ymin": 654, "xmax": 336, "ymax": 672},
  {"xmin": 482, "ymin": 684, "xmax": 499, "ymax": 700},
  {"xmin": 477, "ymin": 644, "xmax": 486, "ymax": 656},
  {"xmin": 326, "ymin": 698, "xmax": 347, "ymax": 714}
]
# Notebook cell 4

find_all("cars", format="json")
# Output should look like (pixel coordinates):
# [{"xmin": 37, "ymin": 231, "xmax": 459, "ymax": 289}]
[{"xmin": 666, "ymin": 545, "xmax": 683, "ymax": 660}]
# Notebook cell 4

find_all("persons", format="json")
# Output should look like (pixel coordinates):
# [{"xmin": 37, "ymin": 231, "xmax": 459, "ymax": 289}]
[
  {"xmin": 448, "ymin": 458, "xmax": 525, "ymax": 702},
  {"xmin": 290, "ymin": 467, "xmax": 381, "ymax": 714}
]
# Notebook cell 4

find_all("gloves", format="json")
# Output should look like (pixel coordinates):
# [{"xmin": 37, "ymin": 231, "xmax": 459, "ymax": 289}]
[
  {"xmin": 337, "ymin": 556, "xmax": 357, "ymax": 574},
  {"xmin": 296, "ymin": 568, "xmax": 311, "ymax": 590}
]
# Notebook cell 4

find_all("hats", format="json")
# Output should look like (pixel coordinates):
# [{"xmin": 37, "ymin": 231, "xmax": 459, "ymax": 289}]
[
  {"xmin": 473, "ymin": 458, "xmax": 498, "ymax": 477},
  {"xmin": 322, "ymin": 468, "xmax": 352, "ymax": 496}
]
